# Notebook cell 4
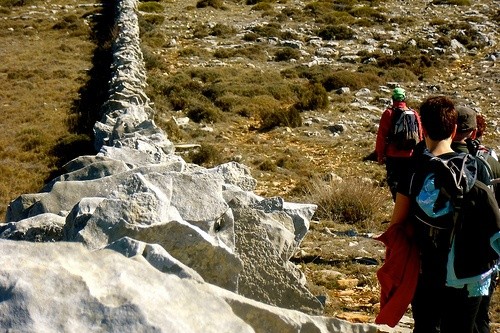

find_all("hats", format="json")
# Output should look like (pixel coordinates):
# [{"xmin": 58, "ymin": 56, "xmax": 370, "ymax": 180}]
[
  {"xmin": 456, "ymin": 106, "xmax": 477, "ymax": 133},
  {"xmin": 392, "ymin": 87, "xmax": 406, "ymax": 101}
]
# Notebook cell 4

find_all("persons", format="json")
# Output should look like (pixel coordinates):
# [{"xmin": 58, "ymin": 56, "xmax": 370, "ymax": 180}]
[
  {"xmin": 389, "ymin": 96, "xmax": 500, "ymax": 333},
  {"xmin": 375, "ymin": 88, "xmax": 422, "ymax": 204}
]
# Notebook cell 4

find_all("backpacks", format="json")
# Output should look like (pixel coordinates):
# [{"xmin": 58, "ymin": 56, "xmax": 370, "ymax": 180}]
[{"xmin": 388, "ymin": 109, "xmax": 418, "ymax": 148}]
